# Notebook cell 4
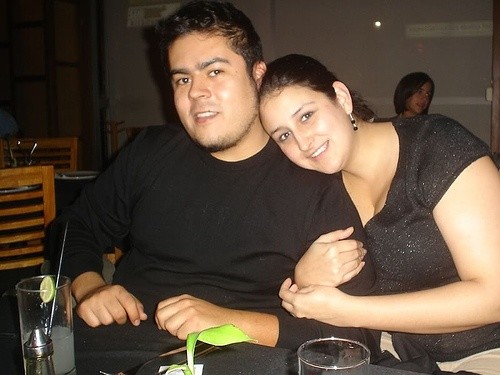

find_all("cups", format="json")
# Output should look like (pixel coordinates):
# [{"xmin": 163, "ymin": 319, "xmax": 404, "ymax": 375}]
[
  {"xmin": 297, "ymin": 338, "xmax": 371, "ymax": 375},
  {"xmin": 16, "ymin": 275, "xmax": 76, "ymax": 375}
]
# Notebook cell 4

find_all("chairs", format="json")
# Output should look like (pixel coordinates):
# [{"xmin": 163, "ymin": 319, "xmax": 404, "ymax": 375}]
[
  {"xmin": 0, "ymin": 135, "xmax": 78, "ymax": 172},
  {"xmin": 0, "ymin": 164, "xmax": 57, "ymax": 270}
]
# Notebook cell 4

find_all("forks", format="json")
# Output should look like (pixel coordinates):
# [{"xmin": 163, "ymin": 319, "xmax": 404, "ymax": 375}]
[{"xmin": 95, "ymin": 340, "xmax": 203, "ymax": 375}]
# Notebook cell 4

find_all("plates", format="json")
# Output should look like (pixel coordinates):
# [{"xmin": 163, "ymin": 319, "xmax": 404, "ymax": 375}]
[{"xmin": 54, "ymin": 171, "xmax": 100, "ymax": 181}]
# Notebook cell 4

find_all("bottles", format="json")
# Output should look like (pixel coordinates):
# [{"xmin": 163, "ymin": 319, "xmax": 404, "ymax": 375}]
[{"xmin": 22, "ymin": 328, "xmax": 57, "ymax": 375}]
